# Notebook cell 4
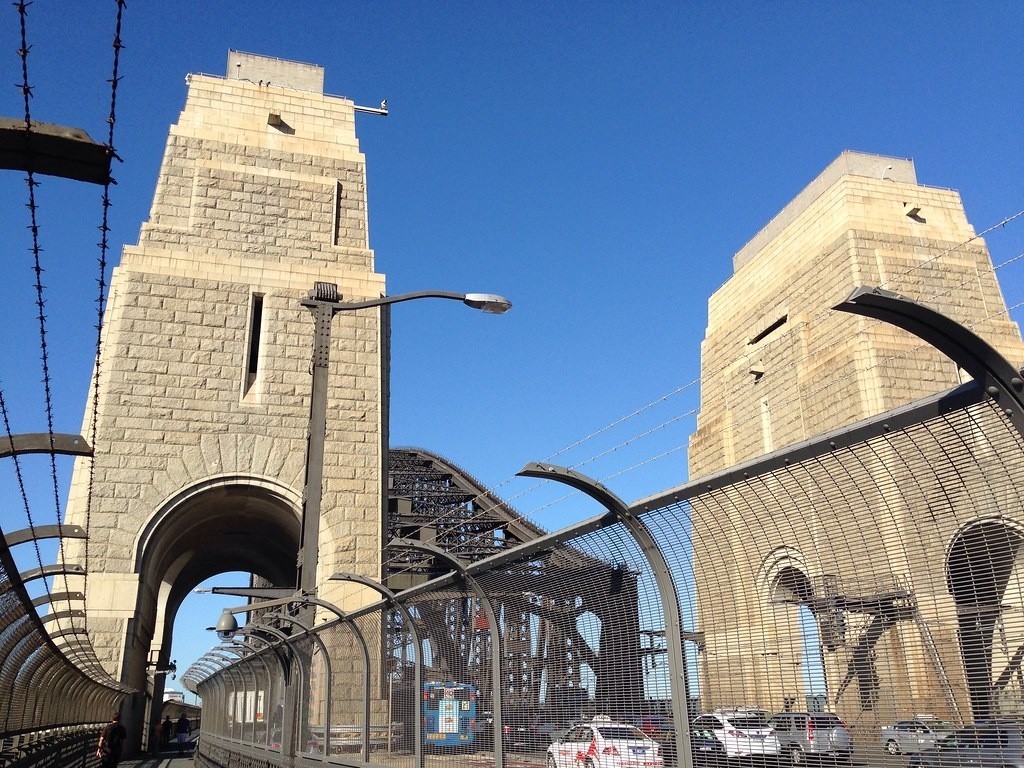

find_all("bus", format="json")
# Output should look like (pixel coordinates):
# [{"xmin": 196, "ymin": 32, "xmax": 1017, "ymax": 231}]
[{"xmin": 391, "ymin": 680, "xmax": 476, "ymax": 753}]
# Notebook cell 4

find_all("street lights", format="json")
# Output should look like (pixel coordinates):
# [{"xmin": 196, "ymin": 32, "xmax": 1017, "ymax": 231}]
[{"xmin": 289, "ymin": 280, "xmax": 512, "ymax": 768}]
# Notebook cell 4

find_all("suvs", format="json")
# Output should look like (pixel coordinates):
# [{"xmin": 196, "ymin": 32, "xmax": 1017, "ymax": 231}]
[{"xmin": 765, "ymin": 712, "xmax": 854, "ymax": 766}]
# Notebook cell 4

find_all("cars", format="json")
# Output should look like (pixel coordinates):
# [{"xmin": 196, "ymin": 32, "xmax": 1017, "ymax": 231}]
[
  {"xmin": 879, "ymin": 717, "xmax": 965, "ymax": 755},
  {"xmin": 908, "ymin": 721, "xmax": 1024, "ymax": 768},
  {"xmin": 475, "ymin": 681, "xmax": 695, "ymax": 750},
  {"xmin": 546, "ymin": 713, "xmax": 665, "ymax": 768},
  {"xmin": 690, "ymin": 711, "xmax": 782, "ymax": 766},
  {"xmin": 650, "ymin": 724, "xmax": 728, "ymax": 768}
]
